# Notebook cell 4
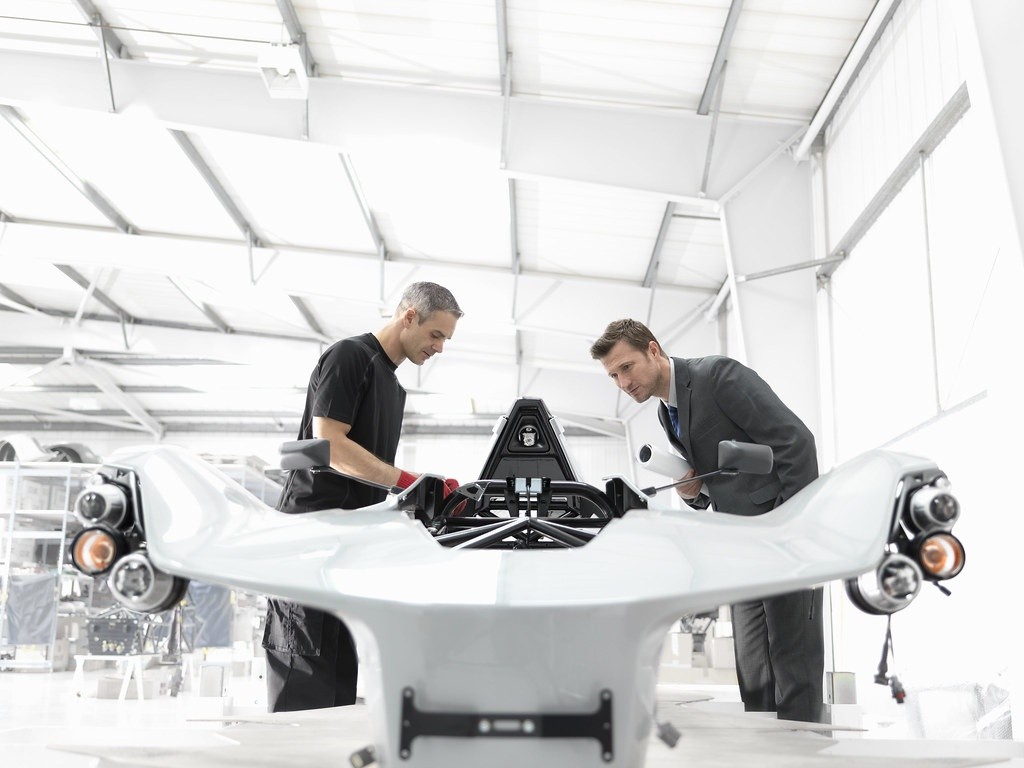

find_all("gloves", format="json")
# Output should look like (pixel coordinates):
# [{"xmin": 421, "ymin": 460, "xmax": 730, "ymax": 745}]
[{"xmin": 396, "ymin": 467, "xmax": 467, "ymax": 516}]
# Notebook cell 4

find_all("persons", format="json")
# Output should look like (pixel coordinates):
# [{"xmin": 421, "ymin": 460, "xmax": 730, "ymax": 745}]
[
  {"xmin": 590, "ymin": 318, "xmax": 827, "ymax": 719},
  {"xmin": 262, "ymin": 282, "xmax": 461, "ymax": 716}
]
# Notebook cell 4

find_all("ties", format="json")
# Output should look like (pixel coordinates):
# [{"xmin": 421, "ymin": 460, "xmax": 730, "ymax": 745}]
[{"xmin": 667, "ymin": 406, "xmax": 681, "ymax": 439}]
